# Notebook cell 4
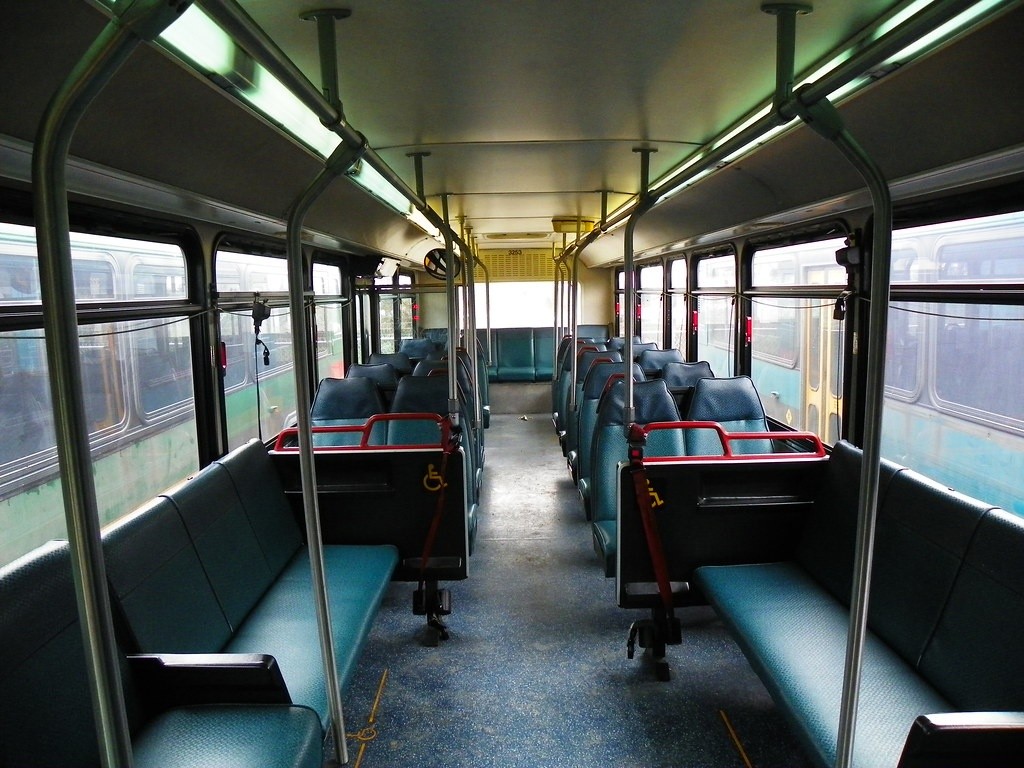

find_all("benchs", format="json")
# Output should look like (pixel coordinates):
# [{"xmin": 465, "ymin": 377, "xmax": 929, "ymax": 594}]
[
  {"xmin": 416, "ymin": 324, "xmax": 611, "ymax": 383},
  {"xmin": 694, "ymin": 438, "xmax": 1024, "ymax": 768},
  {"xmin": 0, "ymin": 439, "xmax": 397, "ymax": 767}
]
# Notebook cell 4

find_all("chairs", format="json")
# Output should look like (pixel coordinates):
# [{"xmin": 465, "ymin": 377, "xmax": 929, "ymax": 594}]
[
  {"xmin": 0, "ymin": 335, "xmax": 297, "ymax": 460},
  {"xmin": 548, "ymin": 333, "xmax": 776, "ymax": 578},
  {"xmin": 310, "ymin": 334, "xmax": 489, "ymax": 559}
]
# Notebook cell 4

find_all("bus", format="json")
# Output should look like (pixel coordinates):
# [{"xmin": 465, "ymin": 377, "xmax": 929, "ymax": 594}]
[
  {"xmin": 671, "ymin": 209, "xmax": 1024, "ymax": 524},
  {"xmin": 0, "ymin": 220, "xmax": 349, "ymax": 571}
]
